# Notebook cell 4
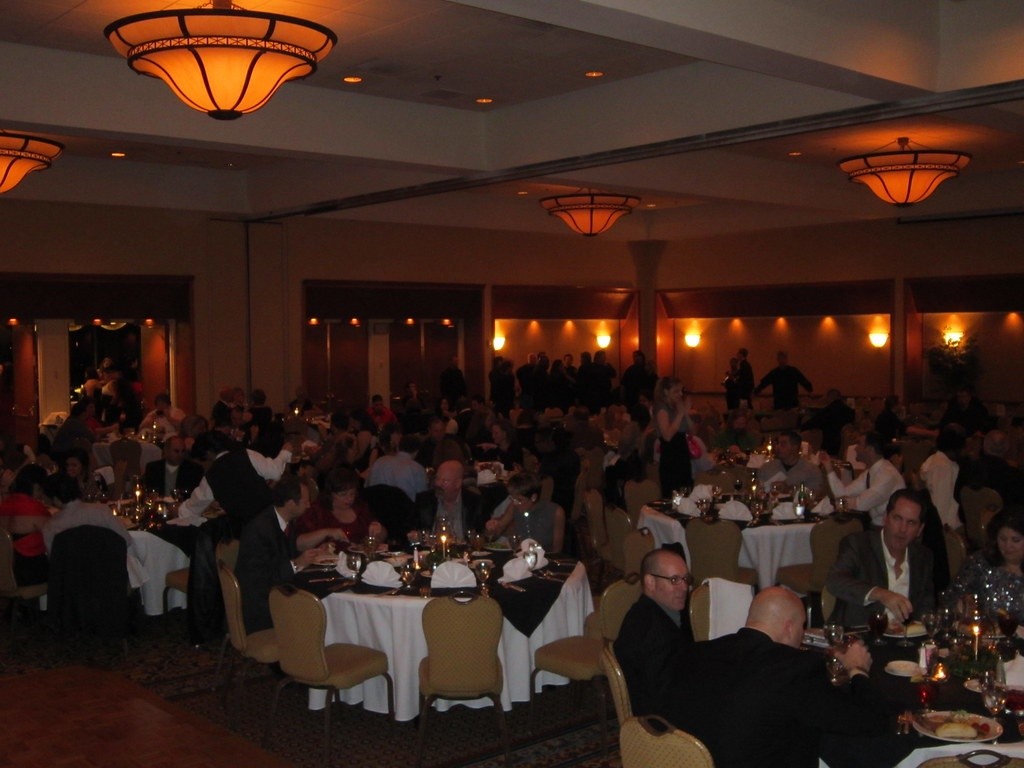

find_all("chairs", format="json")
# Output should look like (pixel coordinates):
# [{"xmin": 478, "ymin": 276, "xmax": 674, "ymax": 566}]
[{"xmin": 0, "ymin": 392, "xmax": 1024, "ymax": 768}]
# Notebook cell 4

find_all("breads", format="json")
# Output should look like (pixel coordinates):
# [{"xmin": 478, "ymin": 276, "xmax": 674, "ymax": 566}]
[
  {"xmin": 935, "ymin": 722, "xmax": 977, "ymax": 738},
  {"xmin": 905, "ymin": 621, "xmax": 927, "ymax": 635}
]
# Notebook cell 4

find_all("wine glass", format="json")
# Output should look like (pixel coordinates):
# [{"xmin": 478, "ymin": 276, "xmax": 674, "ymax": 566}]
[
  {"xmin": 78, "ymin": 482, "xmax": 190, "ymax": 531},
  {"xmin": 347, "ymin": 514, "xmax": 542, "ymax": 600},
  {"xmin": 822, "ymin": 623, "xmax": 858, "ymax": 683},
  {"xmin": 866, "ymin": 600, "xmax": 1024, "ymax": 721},
  {"xmin": 667, "ymin": 434, "xmax": 850, "ymax": 528}
]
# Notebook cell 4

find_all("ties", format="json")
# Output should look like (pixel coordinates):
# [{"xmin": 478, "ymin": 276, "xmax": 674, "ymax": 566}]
[
  {"xmin": 866, "ymin": 473, "xmax": 871, "ymax": 489},
  {"xmin": 284, "ymin": 526, "xmax": 293, "ymax": 541}
]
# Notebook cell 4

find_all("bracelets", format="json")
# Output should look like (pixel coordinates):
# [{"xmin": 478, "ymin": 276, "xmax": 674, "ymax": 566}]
[
  {"xmin": 369, "ymin": 522, "xmax": 381, "ymax": 533},
  {"xmin": 847, "ymin": 665, "xmax": 869, "ymax": 681}
]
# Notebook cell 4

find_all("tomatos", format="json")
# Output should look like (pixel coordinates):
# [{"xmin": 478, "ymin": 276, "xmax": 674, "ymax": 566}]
[{"xmin": 972, "ymin": 723, "xmax": 990, "ymax": 734}]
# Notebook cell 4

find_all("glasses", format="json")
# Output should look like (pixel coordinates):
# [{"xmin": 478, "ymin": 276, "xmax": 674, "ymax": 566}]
[
  {"xmin": 650, "ymin": 573, "xmax": 693, "ymax": 585},
  {"xmin": 509, "ymin": 495, "xmax": 529, "ymax": 505}
]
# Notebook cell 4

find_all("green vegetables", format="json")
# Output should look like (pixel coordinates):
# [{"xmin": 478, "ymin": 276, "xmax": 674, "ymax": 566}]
[{"xmin": 488, "ymin": 543, "xmax": 508, "ymax": 549}]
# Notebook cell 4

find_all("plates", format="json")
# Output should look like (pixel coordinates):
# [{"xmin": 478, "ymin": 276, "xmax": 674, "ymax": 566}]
[
  {"xmin": 912, "ymin": 710, "xmax": 1004, "ymax": 743},
  {"xmin": 348, "ymin": 545, "xmax": 384, "ymax": 554},
  {"xmin": 312, "ymin": 554, "xmax": 340, "ymax": 565},
  {"xmin": 483, "ymin": 543, "xmax": 513, "ymax": 551}
]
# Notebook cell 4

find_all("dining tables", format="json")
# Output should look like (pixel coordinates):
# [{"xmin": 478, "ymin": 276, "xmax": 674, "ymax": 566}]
[
  {"xmin": 636, "ymin": 489, "xmax": 863, "ymax": 600},
  {"xmin": 286, "ymin": 545, "xmax": 594, "ymax": 723},
  {"xmin": 54, "ymin": 496, "xmax": 224, "ymax": 644},
  {"xmin": 791, "ymin": 608, "xmax": 1024, "ymax": 768}
]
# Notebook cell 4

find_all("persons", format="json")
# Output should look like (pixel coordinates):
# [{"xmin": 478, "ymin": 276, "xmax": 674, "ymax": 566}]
[
  {"xmin": 825, "ymin": 489, "xmax": 1024, "ymax": 639},
  {"xmin": 720, "ymin": 346, "xmax": 1024, "ymax": 531},
  {"xmin": 653, "ymin": 376, "xmax": 696, "ymax": 498},
  {"xmin": 0, "ymin": 348, "xmax": 655, "ymax": 697},
  {"xmin": 616, "ymin": 549, "xmax": 873, "ymax": 768}
]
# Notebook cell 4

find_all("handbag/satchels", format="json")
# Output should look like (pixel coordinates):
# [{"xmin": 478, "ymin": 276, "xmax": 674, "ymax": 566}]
[{"xmin": 685, "ymin": 432, "xmax": 701, "ymax": 459}]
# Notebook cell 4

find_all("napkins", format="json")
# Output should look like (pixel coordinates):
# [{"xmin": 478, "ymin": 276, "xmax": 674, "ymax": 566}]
[
  {"xmin": 497, "ymin": 557, "xmax": 533, "ymax": 584},
  {"xmin": 335, "ymin": 551, "xmax": 357, "ymax": 579},
  {"xmin": 430, "ymin": 560, "xmax": 478, "ymax": 589},
  {"xmin": 676, "ymin": 497, "xmax": 701, "ymax": 517},
  {"xmin": 689, "ymin": 484, "xmax": 713, "ymax": 502},
  {"xmin": 771, "ymin": 502, "xmax": 804, "ymax": 520},
  {"xmin": 811, "ymin": 496, "xmax": 834, "ymax": 515},
  {"xmin": 718, "ymin": 500, "xmax": 753, "ymax": 521},
  {"xmin": 361, "ymin": 560, "xmax": 403, "ymax": 588}
]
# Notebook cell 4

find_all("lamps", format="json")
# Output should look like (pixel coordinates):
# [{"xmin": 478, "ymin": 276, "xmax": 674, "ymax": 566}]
[
  {"xmin": 539, "ymin": 194, "xmax": 640, "ymax": 239},
  {"xmin": 103, "ymin": 0, "xmax": 338, "ymax": 121},
  {"xmin": 0, "ymin": 131, "xmax": 65, "ymax": 198},
  {"xmin": 838, "ymin": 136, "xmax": 971, "ymax": 210}
]
